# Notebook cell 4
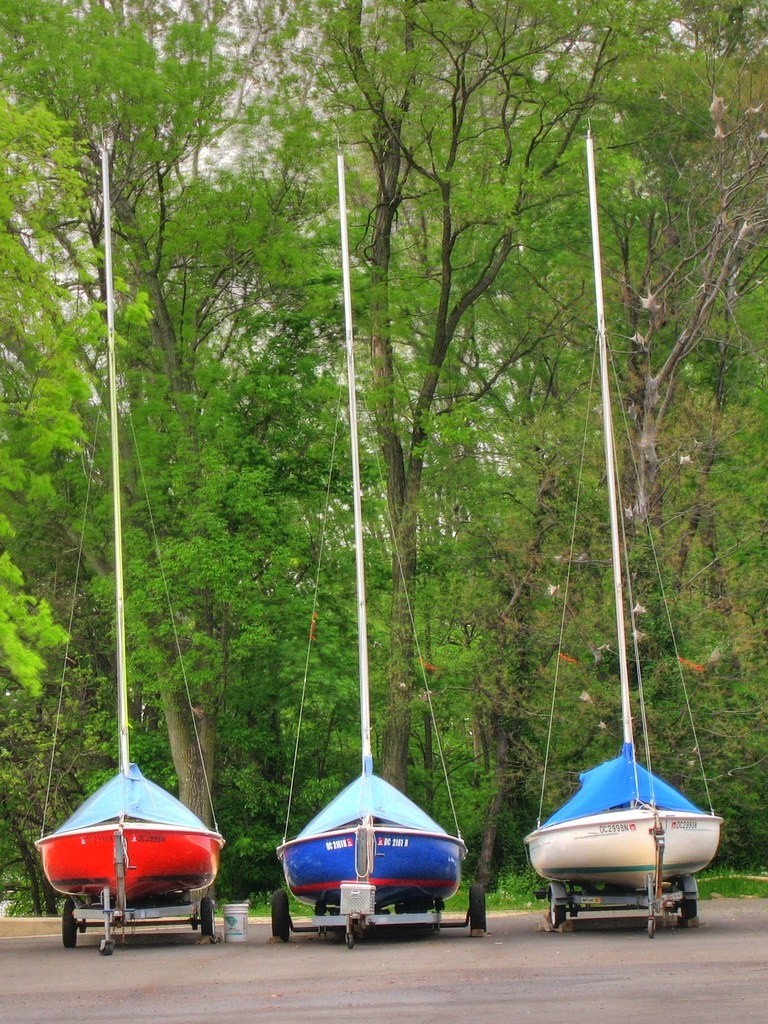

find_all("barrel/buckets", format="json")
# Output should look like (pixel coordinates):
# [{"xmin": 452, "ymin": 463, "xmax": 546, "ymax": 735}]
[{"xmin": 223, "ymin": 904, "xmax": 249, "ymax": 942}]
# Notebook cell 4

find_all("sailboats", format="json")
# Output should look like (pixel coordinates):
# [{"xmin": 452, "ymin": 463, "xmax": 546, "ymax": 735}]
[
  {"xmin": 34, "ymin": 142, "xmax": 227, "ymax": 910},
  {"xmin": 274, "ymin": 147, "xmax": 467, "ymax": 905},
  {"xmin": 520, "ymin": 130, "xmax": 727, "ymax": 885}
]
